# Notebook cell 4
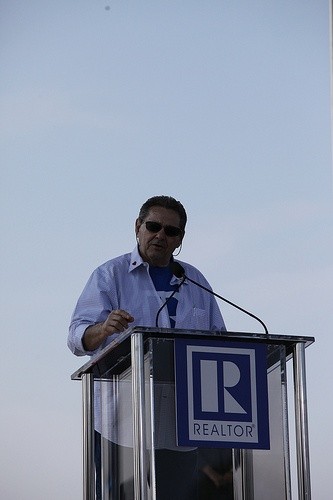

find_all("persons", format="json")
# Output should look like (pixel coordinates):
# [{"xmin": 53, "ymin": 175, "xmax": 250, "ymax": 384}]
[{"xmin": 67, "ymin": 196, "xmax": 228, "ymax": 500}]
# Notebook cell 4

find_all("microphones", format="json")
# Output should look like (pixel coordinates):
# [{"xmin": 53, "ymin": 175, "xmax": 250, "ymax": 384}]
[{"xmin": 156, "ymin": 262, "xmax": 268, "ymax": 334}]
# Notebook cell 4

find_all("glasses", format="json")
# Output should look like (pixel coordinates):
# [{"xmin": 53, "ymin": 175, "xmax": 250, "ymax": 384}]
[{"xmin": 143, "ymin": 221, "xmax": 182, "ymax": 237}]
